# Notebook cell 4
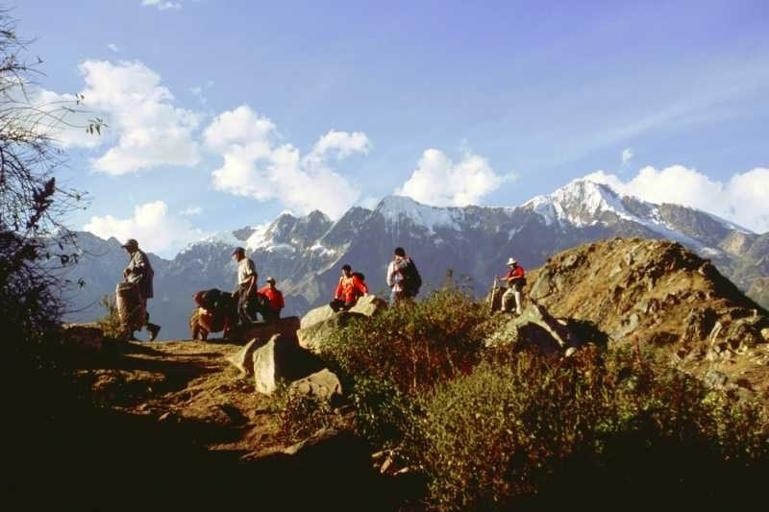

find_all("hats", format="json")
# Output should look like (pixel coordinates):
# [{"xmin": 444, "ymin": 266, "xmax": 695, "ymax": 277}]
[
  {"xmin": 231, "ymin": 247, "xmax": 244, "ymax": 255},
  {"xmin": 121, "ymin": 239, "xmax": 138, "ymax": 247},
  {"xmin": 506, "ymin": 258, "xmax": 518, "ymax": 266},
  {"xmin": 342, "ymin": 265, "xmax": 351, "ymax": 272},
  {"xmin": 266, "ymin": 277, "xmax": 275, "ymax": 285}
]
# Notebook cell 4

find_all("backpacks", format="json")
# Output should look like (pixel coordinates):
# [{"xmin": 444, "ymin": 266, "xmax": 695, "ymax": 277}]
[{"xmin": 395, "ymin": 257, "xmax": 421, "ymax": 291}]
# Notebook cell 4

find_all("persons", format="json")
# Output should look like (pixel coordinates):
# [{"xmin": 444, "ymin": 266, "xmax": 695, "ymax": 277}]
[
  {"xmin": 386, "ymin": 247, "xmax": 411, "ymax": 307},
  {"xmin": 258, "ymin": 277, "xmax": 284, "ymax": 320},
  {"xmin": 230, "ymin": 247, "xmax": 258, "ymax": 324},
  {"xmin": 121, "ymin": 239, "xmax": 160, "ymax": 342},
  {"xmin": 329, "ymin": 264, "xmax": 368, "ymax": 313},
  {"xmin": 496, "ymin": 257, "xmax": 524, "ymax": 317}
]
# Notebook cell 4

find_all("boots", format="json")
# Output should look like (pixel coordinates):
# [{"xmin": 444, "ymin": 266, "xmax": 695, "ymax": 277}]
[{"xmin": 148, "ymin": 323, "xmax": 161, "ymax": 340}]
